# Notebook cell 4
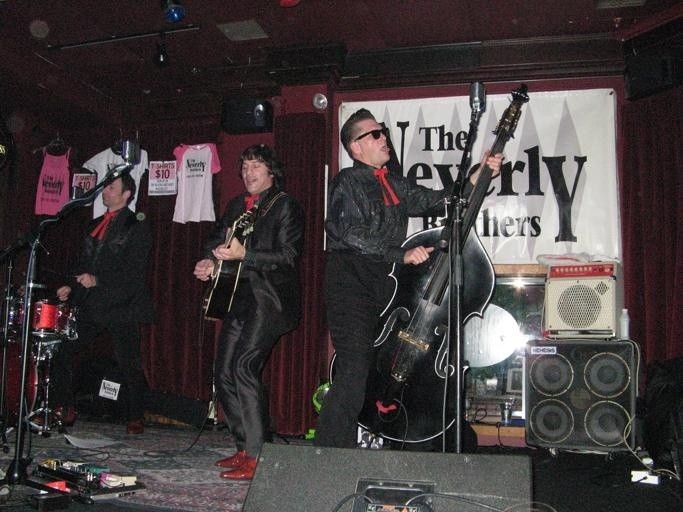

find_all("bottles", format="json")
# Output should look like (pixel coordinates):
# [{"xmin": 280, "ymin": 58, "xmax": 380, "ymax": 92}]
[{"xmin": 618, "ymin": 309, "xmax": 630, "ymax": 340}]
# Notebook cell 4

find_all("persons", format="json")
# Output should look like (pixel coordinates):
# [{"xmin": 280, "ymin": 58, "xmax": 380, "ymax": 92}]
[
  {"xmin": 191, "ymin": 141, "xmax": 308, "ymax": 485},
  {"xmin": 314, "ymin": 107, "xmax": 507, "ymax": 447},
  {"xmin": 28, "ymin": 169, "xmax": 156, "ymax": 440}
]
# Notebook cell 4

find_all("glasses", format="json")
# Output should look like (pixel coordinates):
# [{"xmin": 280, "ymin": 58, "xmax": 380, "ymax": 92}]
[{"xmin": 353, "ymin": 126, "xmax": 386, "ymax": 142}]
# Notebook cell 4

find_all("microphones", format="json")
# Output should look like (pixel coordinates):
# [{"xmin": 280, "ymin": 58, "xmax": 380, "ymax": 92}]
[
  {"xmin": 470, "ymin": 82, "xmax": 486, "ymax": 112},
  {"xmin": 122, "ymin": 140, "xmax": 141, "ymax": 165}
]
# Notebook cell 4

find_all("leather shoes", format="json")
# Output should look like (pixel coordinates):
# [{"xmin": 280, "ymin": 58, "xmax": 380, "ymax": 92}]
[
  {"xmin": 215, "ymin": 451, "xmax": 257, "ymax": 481},
  {"xmin": 32, "ymin": 405, "xmax": 75, "ymax": 426},
  {"xmin": 126, "ymin": 418, "xmax": 143, "ymax": 434}
]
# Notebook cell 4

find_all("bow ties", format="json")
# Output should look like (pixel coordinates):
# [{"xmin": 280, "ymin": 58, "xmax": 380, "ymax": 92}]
[
  {"xmin": 90, "ymin": 211, "xmax": 117, "ymax": 241},
  {"xmin": 373, "ymin": 168, "xmax": 400, "ymax": 206},
  {"xmin": 244, "ymin": 194, "xmax": 259, "ymax": 210}
]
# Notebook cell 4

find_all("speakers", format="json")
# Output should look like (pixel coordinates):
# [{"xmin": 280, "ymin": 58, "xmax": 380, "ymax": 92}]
[
  {"xmin": 544, "ymin": 262, "xmax": 624, "ymax": 342},
  {"xmin": 522, "ymin": 339, "xmax": 642, "ymax": 452},
  {"xmin": 240, "ymin": 443, "xmax": 533, "ymax": 512},
  {"xmin": 221, "ymin": 100, "xmax": 272, "ymax": 134}
]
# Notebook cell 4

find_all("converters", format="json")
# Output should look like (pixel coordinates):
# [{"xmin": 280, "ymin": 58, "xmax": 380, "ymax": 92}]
[
  {"xmin": 32, "ymin": 492, "xmax": 72, "ymax": 510},
  {"xmin": 631, "ymin": 470, "xmax": 660, "ymax": 484}
]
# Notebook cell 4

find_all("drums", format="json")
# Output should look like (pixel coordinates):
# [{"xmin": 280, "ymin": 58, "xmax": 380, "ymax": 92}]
[{"xmin": 14, "ymin": 299, "xmax": 76, "ymax": 342}]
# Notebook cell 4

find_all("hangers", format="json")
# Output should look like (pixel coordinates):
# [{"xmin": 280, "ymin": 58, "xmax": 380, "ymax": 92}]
[{"xmin": 31, "ymin": 127, "xmax": 72, "ymax": 154}]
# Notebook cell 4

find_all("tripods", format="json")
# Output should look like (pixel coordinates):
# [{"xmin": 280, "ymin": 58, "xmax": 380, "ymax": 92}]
[{"xmin": 0, "ymin": 165, "xmax": 95, "ymax": 506}]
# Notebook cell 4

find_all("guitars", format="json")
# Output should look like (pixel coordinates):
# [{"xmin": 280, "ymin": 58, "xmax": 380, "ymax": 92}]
[{"xmin": 204, "ymin": 206, "xmax": 256, "ymax": 320}]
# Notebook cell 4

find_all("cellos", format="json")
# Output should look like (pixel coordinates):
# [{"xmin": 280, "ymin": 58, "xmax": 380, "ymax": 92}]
[{"xmin": 329, "ymin": 82, "xmax": 530, "ymax": 443}]
